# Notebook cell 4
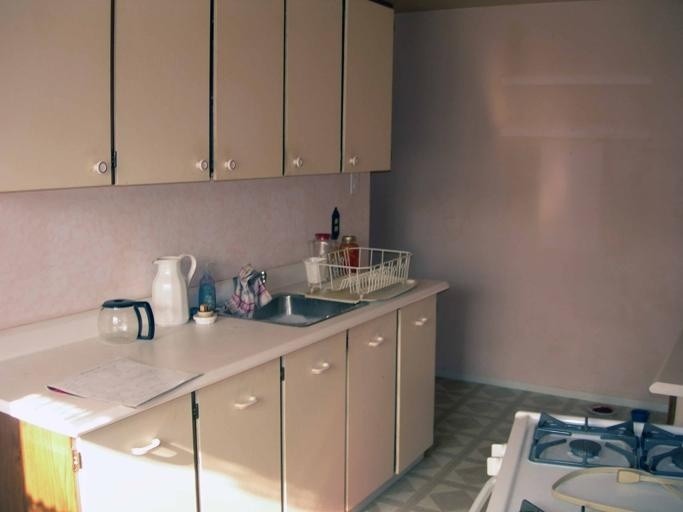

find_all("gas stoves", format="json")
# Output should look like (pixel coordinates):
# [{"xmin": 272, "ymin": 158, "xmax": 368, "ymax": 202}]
[{"xmin": 483, "ymin": 406, "xmax": 683, "ymax": 512}]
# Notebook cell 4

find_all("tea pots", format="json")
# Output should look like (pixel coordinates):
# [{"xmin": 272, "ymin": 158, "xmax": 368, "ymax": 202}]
[
  {"xmin": 96, "ymin": 300, "xmax": 154, "ymax": 343},
  {"xmin": 150, "ymin": 253, "xmax": 195, "ymax": 327}
]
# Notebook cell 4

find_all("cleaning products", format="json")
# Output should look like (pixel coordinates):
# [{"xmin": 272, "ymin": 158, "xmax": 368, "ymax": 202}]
[{"xmin": 198, "ymin": 270, "xmax": 217, "ymax": 312}]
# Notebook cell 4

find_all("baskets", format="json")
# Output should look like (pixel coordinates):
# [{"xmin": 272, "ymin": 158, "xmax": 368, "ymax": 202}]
[{"xmin": 310, "ymin": 247, "xmax": 414, "ymax": 295}]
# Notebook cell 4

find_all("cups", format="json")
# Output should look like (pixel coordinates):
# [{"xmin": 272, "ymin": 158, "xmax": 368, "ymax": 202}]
[{"xmin": 302, "ymin": 257, "xmax": 326, "ymax": 288}]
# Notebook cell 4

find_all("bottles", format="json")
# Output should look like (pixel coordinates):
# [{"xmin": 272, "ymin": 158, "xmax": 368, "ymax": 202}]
[
  {"xmin": 338, "ymin": 235, "xmax": 359, "ymax": 273},
  {"xmin": 311, "ymin": 233, "xmax": 333, "ymax": 256}
]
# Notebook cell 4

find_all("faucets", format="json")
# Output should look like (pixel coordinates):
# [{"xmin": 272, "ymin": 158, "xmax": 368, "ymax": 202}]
[{"xmin": 232, "ymin": 270, "xmax": 267, "ymax": 293}]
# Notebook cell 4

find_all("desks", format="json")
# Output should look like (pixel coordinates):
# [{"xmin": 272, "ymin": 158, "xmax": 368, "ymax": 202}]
[{"xmin": 649, "ymin": 330, "xmax": 683, "ymax": 427}]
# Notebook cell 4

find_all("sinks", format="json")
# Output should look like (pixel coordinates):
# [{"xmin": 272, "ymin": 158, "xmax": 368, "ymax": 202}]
[{"xmin": 217, "ymin": 291, "xmax": 370, "ymax": 328}]
